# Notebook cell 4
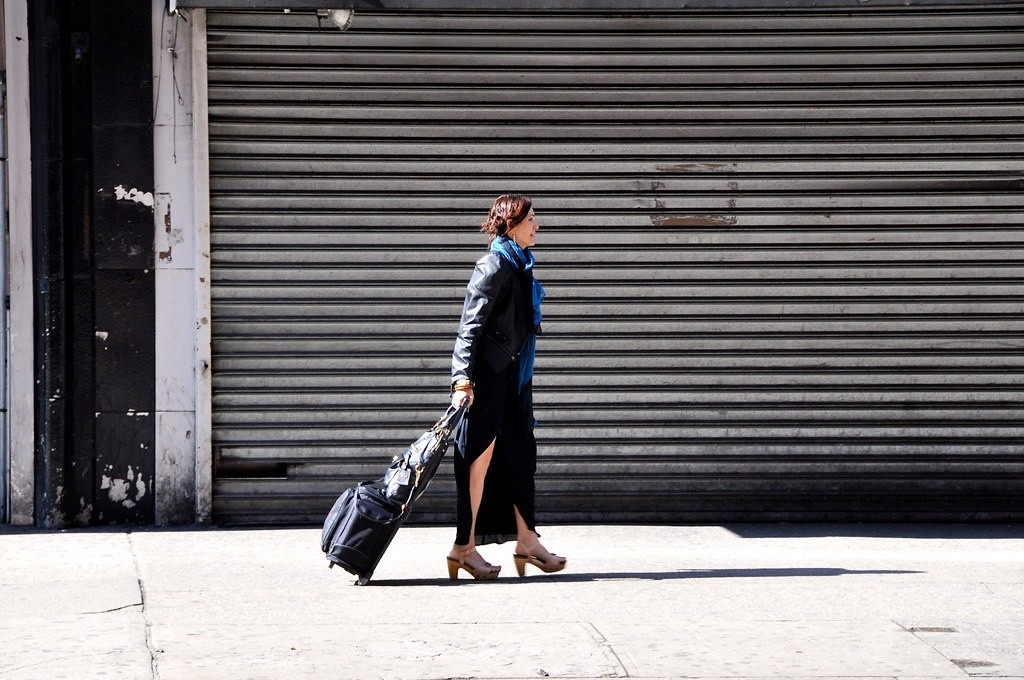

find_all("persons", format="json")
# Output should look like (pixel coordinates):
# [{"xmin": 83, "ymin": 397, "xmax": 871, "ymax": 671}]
[{"xmin": 446, "ymin": 194, "xmax": 568, "ymax": 580}]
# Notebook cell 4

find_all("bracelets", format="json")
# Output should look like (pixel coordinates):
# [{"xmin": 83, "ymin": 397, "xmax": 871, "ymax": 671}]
[{"xmin": 453, "ymin": 384, "xmax": 473, "ymax": 391}]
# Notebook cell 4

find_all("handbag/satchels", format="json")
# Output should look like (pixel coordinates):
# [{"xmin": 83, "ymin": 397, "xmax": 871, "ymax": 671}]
[{"xmin": 383, "ymin": 398, "xmax": 468, "ymax": 504}]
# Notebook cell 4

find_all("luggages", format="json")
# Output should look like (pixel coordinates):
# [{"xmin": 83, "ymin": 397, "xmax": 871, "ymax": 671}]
[{"xmin": 321, "ymin": 395, "xmax": 468, "ymax": 585}]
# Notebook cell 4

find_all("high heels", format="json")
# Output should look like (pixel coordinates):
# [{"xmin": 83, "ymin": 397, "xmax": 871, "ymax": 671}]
[
  {"xmin": 513, "ymin": 539, "xmax": 566, "ymax": 577},
  {"xmin": 446, "ymin": 540, "xmax": 501, "ymax": 581}
]
